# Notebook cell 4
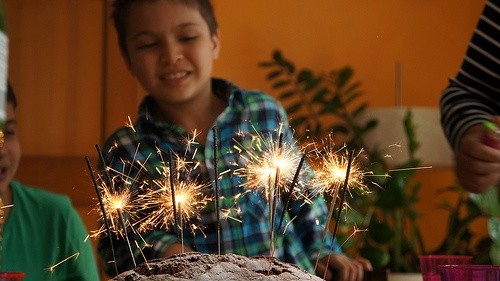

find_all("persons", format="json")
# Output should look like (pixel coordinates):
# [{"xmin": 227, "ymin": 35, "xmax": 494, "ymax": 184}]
[
  {"xmin": 0, "ymin": 80, "xmax": 100, "ymax": 281},
  {"xmin": 97, "ymin": 0, "xmax": 372, "ymax": 281},
  {"xmin": 439, "ymin": 0, "xmax": 500, "ymax": 193}
]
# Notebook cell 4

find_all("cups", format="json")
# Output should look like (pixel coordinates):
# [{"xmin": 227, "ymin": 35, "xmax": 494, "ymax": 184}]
[
  {"xmin": 418, "ymin": 255, "xmax": 473, "ymax": 281},
  {"xmin": 437, "ymin": 265, "xmax": 500, "ymax": 281}
]
